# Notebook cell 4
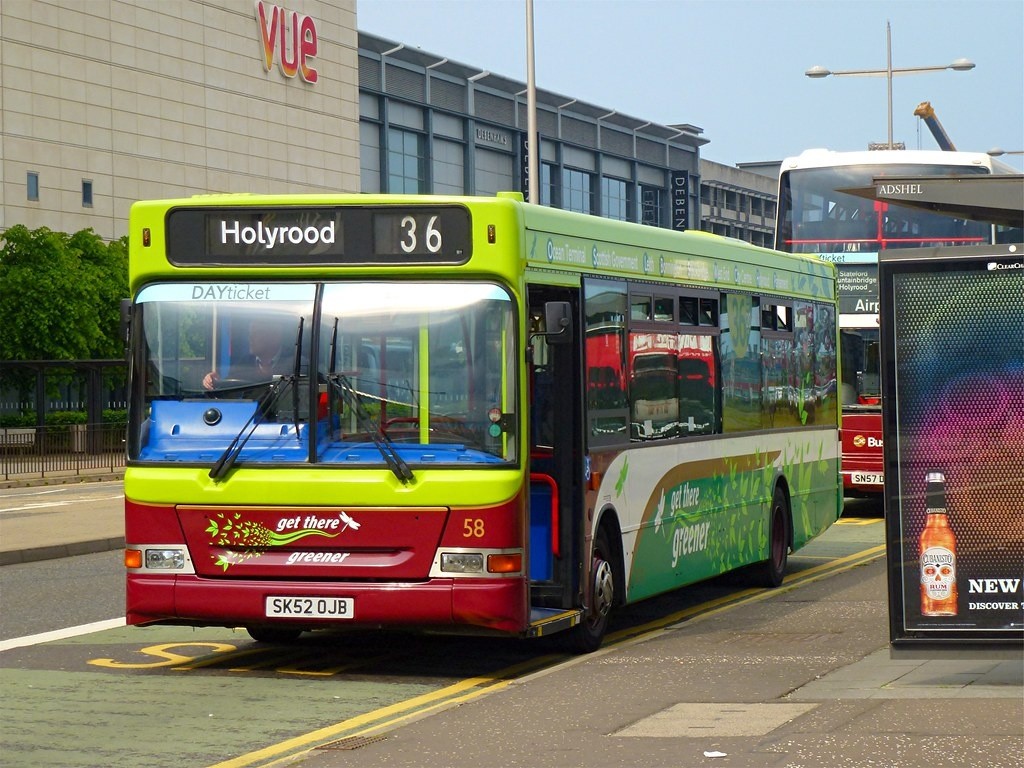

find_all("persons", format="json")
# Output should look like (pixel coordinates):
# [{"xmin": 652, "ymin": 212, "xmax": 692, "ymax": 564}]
[{"xmin": 202, "ymin": 316, "xmax": 310, "ymax": 393}]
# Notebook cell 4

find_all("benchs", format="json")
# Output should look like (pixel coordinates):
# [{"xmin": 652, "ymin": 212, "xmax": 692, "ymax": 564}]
[{"xmin": 0, "ymin": 428, "xmax": 37, "ymax": 455}]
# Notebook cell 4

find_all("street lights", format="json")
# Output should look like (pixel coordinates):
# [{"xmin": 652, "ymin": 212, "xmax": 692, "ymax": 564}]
[{"xmin": 805, "ymin": 17, "xmax": 977, "ymax": 152}]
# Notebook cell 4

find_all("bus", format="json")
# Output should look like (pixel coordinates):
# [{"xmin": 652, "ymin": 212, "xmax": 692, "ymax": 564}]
[
  {"xmin": 771, "ymin": 147, "xmax": 1024, "ymax": 504},
  {"xmin": 629, "ymin": 351, "xmax": 681, "ymax": 445},
  {"xmin": 121, "ymin": 188, "xmax": 845, "ymax": 656}
]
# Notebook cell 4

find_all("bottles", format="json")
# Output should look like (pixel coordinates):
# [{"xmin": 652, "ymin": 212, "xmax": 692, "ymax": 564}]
[{"xmin": 917, "ymin": 467, "xmax": 960, "ymax": 619}]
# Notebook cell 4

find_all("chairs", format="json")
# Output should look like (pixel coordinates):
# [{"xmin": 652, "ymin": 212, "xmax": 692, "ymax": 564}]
[{"xmin": 588, "ymin": 355, "xmax": 712, "ymax": 442}]
